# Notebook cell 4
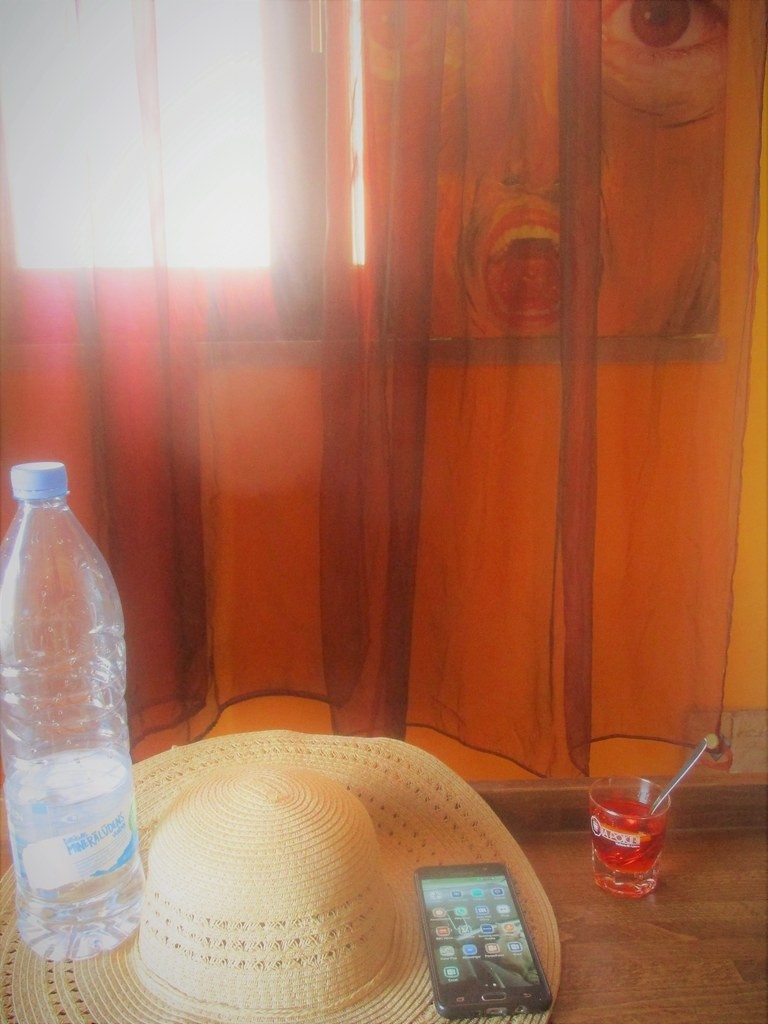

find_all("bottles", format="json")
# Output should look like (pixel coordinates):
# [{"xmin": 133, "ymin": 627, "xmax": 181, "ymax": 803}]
[{"xmin": 0, "ymin": 462, "xmax": 145, "ymax": 961}]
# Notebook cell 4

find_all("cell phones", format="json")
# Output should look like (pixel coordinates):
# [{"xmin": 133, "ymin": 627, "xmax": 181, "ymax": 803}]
[{"xmin": 412, "ymin": 862, "xmax": 552, "ymax": 1019}]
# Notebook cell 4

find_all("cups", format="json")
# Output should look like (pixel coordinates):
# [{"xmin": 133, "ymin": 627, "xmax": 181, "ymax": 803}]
[{"xmin": 589, "ymin": 775, "xmax": 670, "ymax": 899}]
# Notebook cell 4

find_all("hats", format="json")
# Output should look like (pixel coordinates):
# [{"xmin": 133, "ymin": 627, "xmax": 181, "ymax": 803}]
[{"xmin": 0, "ymin": 729, "xmax": 561, "ymax": 1024}]
